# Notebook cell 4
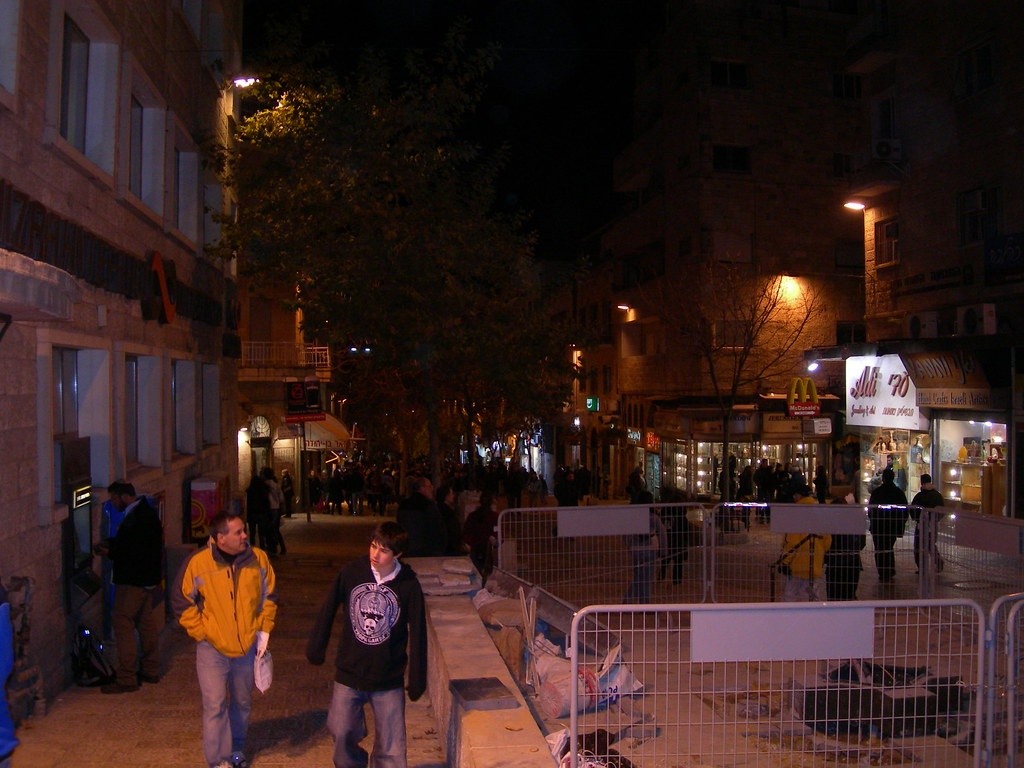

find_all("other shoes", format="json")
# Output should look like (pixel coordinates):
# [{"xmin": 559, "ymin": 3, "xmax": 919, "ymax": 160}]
[
  {"xmin": 935, "ymin": 559, "xmax": 943, "ymax": 573},
  {"xmin": 103, "ymin": 681, "xmax": 139, "ymax": 694},
  {"xmin": 283, "ymin": 515, "xmax": 291, "ymax": 518},
  {"xmin": 136, "ymin": 670, "xmax": 159, "ymax": 683},
  {"xmin": 232, "ymin": 751, "xmax": 246, "ymax": 768},
  {"xmin": 915, "ymin": 571, "xmax": 919, "ymax": 574}
]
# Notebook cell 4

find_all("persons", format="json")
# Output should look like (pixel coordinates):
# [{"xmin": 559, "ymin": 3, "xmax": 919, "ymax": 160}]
[
  {"xmin": 93, "ymin": 480, "xmax": 166, "ymax": 693},
  {"xmin": 170, "ymin": 507, "xmax": 278, "ymax": 768},
  {"xmin": 245, "ymin": 451, "xmax": 947, "ymax": 603},
  {"xmin": 305, "ymin": 519, "xmax": 427, "ymax": 768}
]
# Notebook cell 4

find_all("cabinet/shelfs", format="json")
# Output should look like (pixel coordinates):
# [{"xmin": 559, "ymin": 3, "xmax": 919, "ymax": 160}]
[
  {"xmin": 859, "ymin": 426, "xmax": 911, "ymax": 532},
  {"xmin": 940, "ymin": 460, "xmax": 992, "ymax": 514},
  {"xmin": 909, "ymin": 461, "xmax": 930, "ymax": 493}
]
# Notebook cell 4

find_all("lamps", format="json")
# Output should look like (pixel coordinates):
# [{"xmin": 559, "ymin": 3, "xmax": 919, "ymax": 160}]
[
  {"xmin": 843, "ymin": 200, "xmax": 866, "ymax": 212},
  {"xmin": 617, "ymin": 305, "xmax": 630, "ymax": 313},
  {"xmin": 227, "ymin": 73, "xmax": 261, "ymax": 91}
]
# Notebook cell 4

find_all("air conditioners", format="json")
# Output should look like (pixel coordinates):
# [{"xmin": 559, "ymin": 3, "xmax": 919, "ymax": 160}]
[
  {"xmin": 956, "ymin": 302, "xmax": 996, "ymax": 335},
  {"xmin": 900, "ymin": 310, "xmax": 938, "ymax": 338},
  {"xmin": 864, "ymin": 136, "xmax": 903, "ymax": 162}
]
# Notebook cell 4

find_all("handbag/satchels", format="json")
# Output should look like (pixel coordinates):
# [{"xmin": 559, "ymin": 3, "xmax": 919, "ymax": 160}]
[
  {"xmin": 254, "ymin": 631, "xmax": 273, "ymax": 694},
  {"xmin": 71, "ymin": 624, "xmax": 116, "ymax": 688}
]
